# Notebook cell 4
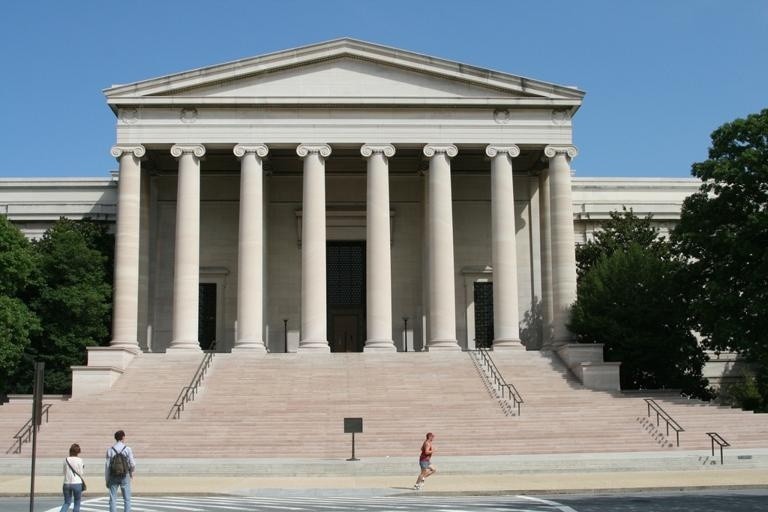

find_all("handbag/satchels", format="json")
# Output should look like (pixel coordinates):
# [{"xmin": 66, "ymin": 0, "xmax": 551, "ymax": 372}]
[{"xmin": 82, "ymin": 482, "xmax": 86, "ymax": 490}]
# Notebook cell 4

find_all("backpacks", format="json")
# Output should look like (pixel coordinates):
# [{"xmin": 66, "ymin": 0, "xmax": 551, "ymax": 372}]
[{"xmin": 111, "ymin": 446, "xmax": 128, "ymax": 479}]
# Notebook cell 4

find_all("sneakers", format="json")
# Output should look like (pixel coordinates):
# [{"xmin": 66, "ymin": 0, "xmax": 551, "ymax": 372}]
[
  {"xmin": 420, "ymin": 477, "xmax": 424, "ymax": 485},
  {"xmin": 413, "ymin": 485, "xmax": 423, "ymax": 490}
]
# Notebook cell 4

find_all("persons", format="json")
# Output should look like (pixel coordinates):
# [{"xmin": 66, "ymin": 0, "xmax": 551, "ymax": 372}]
[
  {"xmin": 60, "ymin": 444, "xmax": 85, "ymax": 511},
  {"xmin": 413, "ymin": 431, "xmax": 436, "ymax": 490},
  {"xmin": 105, "ymin": 430, "xmax": 136, "ymax": 512}
]
